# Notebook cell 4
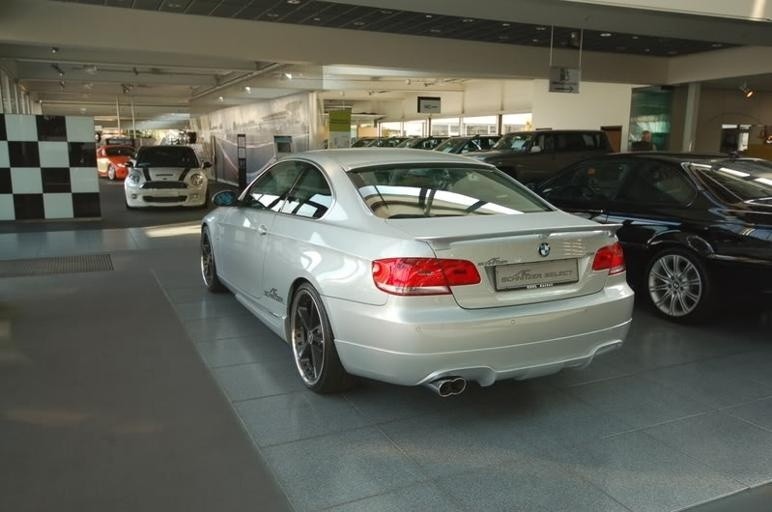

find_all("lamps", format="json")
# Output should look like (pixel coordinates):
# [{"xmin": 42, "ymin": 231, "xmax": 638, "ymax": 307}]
[
  {"xmin": 209, "ymin": 66, "xmax": 296, "ymax": 104},
  {"xmin": 736, "ymin": 80, "xmax": 755, "ymax": 101},
  {"xmin": 402, "ymin": 79, "xmax": 411, "ymax": 86},
  {"xmin": 132, "ymin": 66, "xmax": 138, "ymax": 73},
  {"xmin": 123, "ymin": 84, "xmax": 133, "ymax": 95},
  {"xmin": 58, "ymin": 70, "xmax": 65, "ymax": 77},
  {"xmin": 51, "ymin": 47, "xmax": 59, "ymax": 53}
]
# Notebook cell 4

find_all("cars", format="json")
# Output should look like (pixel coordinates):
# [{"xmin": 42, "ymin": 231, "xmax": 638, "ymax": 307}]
[
  {"xmin": 124, "ymin": 144, "xmax": 213, "ymax": 210},
  {"xmin": 96, "ymin": 144, "xmax": 137, "ymax": 181},
  {"xmin": 200, "ymin": 147, "xmax": 634, "ymax": 396},
  {"xmin": 524, "ymin": 151, "xmax": 771, "ymax": 322},
  {"xmin": 318, "ymin": 130, "xmax": 616, "ymax": 185},
  {"xmin": 125, "ymin": 129, "xmax": 144, "ymax": 137}
]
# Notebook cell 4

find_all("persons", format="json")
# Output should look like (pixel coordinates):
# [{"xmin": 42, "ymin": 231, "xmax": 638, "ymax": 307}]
[{"xmin": 630, "ymin": 130, "xmax": 656, "ymax": 151}]
